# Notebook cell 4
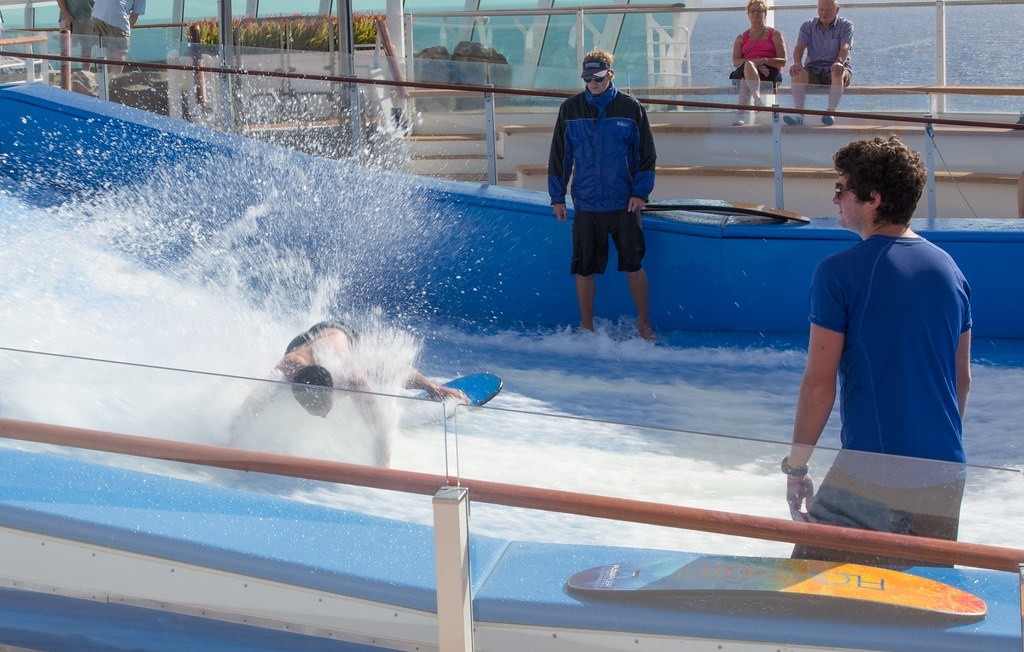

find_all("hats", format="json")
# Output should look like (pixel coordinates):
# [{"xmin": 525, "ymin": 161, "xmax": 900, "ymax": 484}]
[{"xmin": 582, "ymin": 59, "xmax": 610, "ymax": 77}]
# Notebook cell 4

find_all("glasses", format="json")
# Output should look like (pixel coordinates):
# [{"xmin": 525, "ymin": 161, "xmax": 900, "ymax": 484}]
[
  {"xmin": 584, "ymin": 76, "xmax": 604, "ymax": 82},
  {"xmin": 748, "ymin": 8, "xmax": 763, "ymax": 12},
  {"xmin": 834, "ymin": 183, "xmax": 856, "ymax": 198}
]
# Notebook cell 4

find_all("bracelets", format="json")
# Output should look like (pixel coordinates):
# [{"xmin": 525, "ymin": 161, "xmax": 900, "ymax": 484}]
[{"xmin": 837, "ymin": 59, "xmax": 843, "ymax": 64}]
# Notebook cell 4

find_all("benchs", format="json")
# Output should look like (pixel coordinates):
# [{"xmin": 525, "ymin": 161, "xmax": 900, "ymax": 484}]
[
  {"xmin": 498, "ymin": 122, "xmax": 1024, "ymax": 161},
  {"xmin": 519, "ymin": 163, "xmax": 1024, "ymax": 219},
  {"xmin": 405, "ymin": 86, "xmax": 1023, "ymax": 136}
]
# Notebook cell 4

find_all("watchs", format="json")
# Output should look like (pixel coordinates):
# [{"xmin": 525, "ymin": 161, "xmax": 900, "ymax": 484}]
[{"xmin": 780, "ymin": 456, "xmax": 809, "ymax": 477}]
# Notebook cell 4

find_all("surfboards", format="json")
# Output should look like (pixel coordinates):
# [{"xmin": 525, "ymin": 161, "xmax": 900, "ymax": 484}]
[
  {"xmin": 640, "ymin": 197, "xmax": 812, "ymax": 225},
  {"xmin": 346, "ymin": 371, "xmax": 504, "ymax": 431},
  {"xmin": 565, "ymin": 556, "xmax": 987, "ymax": 618}
]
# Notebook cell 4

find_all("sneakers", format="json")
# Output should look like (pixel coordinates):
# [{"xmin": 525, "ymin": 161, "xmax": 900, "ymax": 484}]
[
  {"xmin": 733, "ymin": 115, "xmax": 743, "ymax": 125},
  {"xmin": 752, "ymin": 97, "xmax": 765, "ymax": 110},
  {"xmin": 784, "ymin": 114, "xmax": 806, "ymax": 126},
  {"xmin": 821, "ymin": 114, "xmax": 835, "ymax": 127}
]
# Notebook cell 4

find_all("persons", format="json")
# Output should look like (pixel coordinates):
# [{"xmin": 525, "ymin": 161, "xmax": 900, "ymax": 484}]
[
  {"xmin": 732, "ymin": 0, "xmax": 855, "ymax": 126},
  {"xmin": 547, "ymin": 50, "xmax": 657, "ymax": 341},
  {"xmin": 228, "ymin": 318, "xmax": 473, "ymax": 469},
  {"xmin": 56, "ymin": 0, "xmax": 146, "ymax": 75},
  {"xmin": 786, "ymin": 136, "xmax": 973, "ymax": 569}
]
